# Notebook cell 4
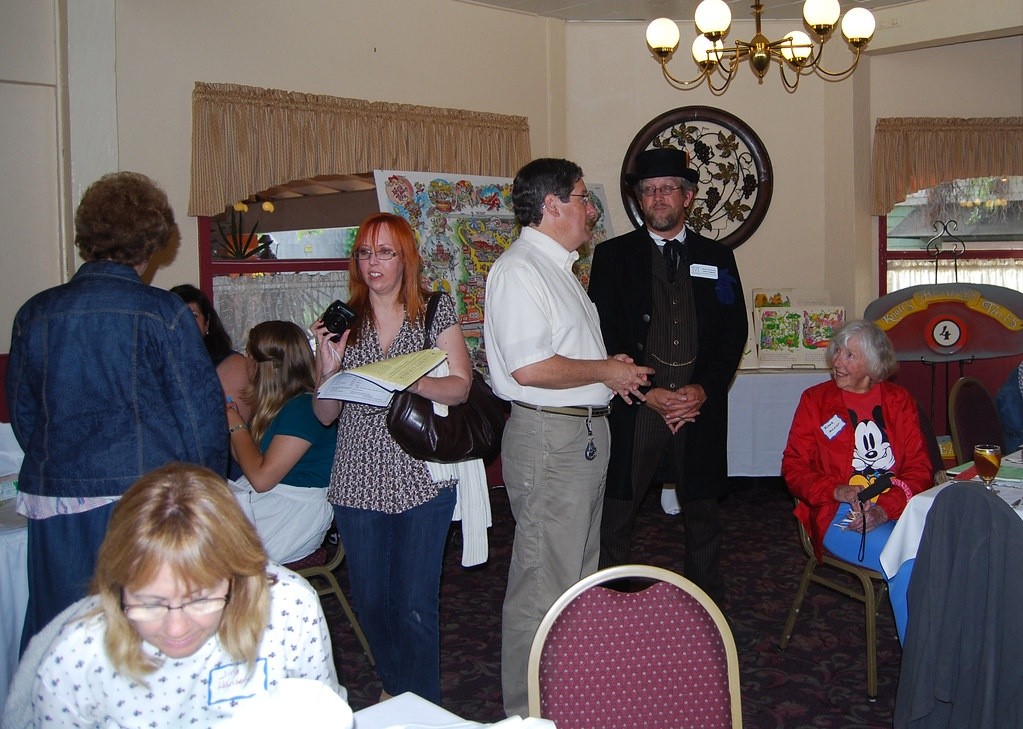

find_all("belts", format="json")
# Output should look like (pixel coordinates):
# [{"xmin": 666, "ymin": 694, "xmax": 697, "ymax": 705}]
[{"xmin": 512, "ymin": 399, "xmax": 612, "ymax": 417}]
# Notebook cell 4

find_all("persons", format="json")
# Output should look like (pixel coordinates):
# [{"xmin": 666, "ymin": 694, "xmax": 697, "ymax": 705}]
[
  {"xmin": 228, "ymin": 321, "xmax": 339, "ymax": 562},
  {"xmin": 169, "ymin": 284, "xmax": 252, "ymax": 426},
  {"xmin": 32, "ymin": 461, "xmax": 347, "ymax": 729},
  {"xmin": 6, "ymin": 171, "xmax": 228, "ymax": 651},
  {"xmin": 312, "ymin": 213, "xmax": 474, "ymax": 706},
  {"xmin": 781, "ymin": 320, "xmax": 933, "ymax": 646},
  {"xmin": 588, "ymin": 148, "xmax": 748, "ymax": 627},
  {"xmin": 483, "ymin": 159, "xmax": 657, "ymax": 720},
  {"xmin": 996, "ymin": 360, "xmax": 1023, "ymax": 456}
]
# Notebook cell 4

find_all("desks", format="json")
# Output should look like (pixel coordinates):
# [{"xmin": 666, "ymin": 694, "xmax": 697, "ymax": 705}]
[
  {"xmin": 353, "ymin": 690, "xmax": 468, "ymax": 729},
  {"xmin": 879, "ymin": 449, "xmax": 1023, "ymax": 581},
  {"xmin": 0, "ymin": 422, "xmax": 30, "ymax": 708}
]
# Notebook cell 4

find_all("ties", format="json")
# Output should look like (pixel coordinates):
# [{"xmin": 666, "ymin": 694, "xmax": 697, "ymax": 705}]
[{"xmin": 662, "ymin": 238, "xmax": 681, "ymax": 285}]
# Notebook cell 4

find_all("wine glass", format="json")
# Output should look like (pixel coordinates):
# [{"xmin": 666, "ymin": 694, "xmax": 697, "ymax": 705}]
[{"xmin": 974, "ymin": 445, "xmax": 1001, "ymax": 494}]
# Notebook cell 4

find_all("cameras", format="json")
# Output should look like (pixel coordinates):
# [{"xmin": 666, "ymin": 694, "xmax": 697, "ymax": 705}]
[{"xmin": 318, "ymin": 299, "xmax": 358, "ymax": 343}]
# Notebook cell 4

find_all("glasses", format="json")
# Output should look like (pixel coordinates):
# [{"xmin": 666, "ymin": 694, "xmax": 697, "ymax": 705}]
[
  {"xmin": 639, "ymin": 185, "xmax": 684, "ymax": 197},
  {"xmin": 542, "ymin": 192, "xmax": 592, "ymax": 207},
  {"xmin": 351, "ymin": 247, "xmax": 402, "ymax": 261},
  {"xmin": 117, "ymin": 577, "xmax": 237, "ymax": 622}
]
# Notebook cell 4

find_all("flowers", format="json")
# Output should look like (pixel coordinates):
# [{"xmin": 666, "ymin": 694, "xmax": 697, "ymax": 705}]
[{"xmin": 212, "ymin": 202, "xmax": 274, "ymax": 259}]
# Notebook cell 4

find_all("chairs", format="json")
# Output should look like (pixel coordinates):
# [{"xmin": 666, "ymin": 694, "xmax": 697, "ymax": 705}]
[
  {"xmin": 945, "ymin": 375, "xmax": 1008, "ymax": 464},
  {"xmin": 895, "ymin": 482, "xmax": 1023, "ymax": 729},
  {"xmin": 916, "ymin": 403, "xmax": 950, "ymax": 487},
  {"xmin": 777, "ymin": 499, "xmax": 890, "ymax": 702},
  {"xmin": 282, "ymin": 519, "xmax": 376, "ymax": 667},
  {"xmin": 524, "ymin": 566, "xmax": 745, "ymax": 729}
]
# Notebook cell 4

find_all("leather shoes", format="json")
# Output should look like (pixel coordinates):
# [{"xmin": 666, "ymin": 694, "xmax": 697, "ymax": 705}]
[{"xmin": 714, "ymin": 613, "xmax": 757, "ymax": 653}]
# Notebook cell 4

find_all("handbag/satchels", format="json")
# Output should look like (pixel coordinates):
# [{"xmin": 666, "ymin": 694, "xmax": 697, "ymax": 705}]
[{"xmin": 386, "ymin": 290, "xmax": 501, "ymax": 464}]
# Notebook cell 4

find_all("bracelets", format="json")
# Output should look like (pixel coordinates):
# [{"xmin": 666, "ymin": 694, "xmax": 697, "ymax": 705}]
[{"xmin": 230, "ymin": 425, "xmax": 246, "ymax": 432}]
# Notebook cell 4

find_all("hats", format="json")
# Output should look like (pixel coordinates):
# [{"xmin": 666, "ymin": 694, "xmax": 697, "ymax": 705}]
[{"xmin": 622, "ymin": 150, "xmax": 699, "ymax": 184}]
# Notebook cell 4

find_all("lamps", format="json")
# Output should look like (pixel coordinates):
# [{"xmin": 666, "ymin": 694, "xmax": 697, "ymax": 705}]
[{"xmin": 645, "ymin": 0, "xmax": 877, "ymax": 90}]
